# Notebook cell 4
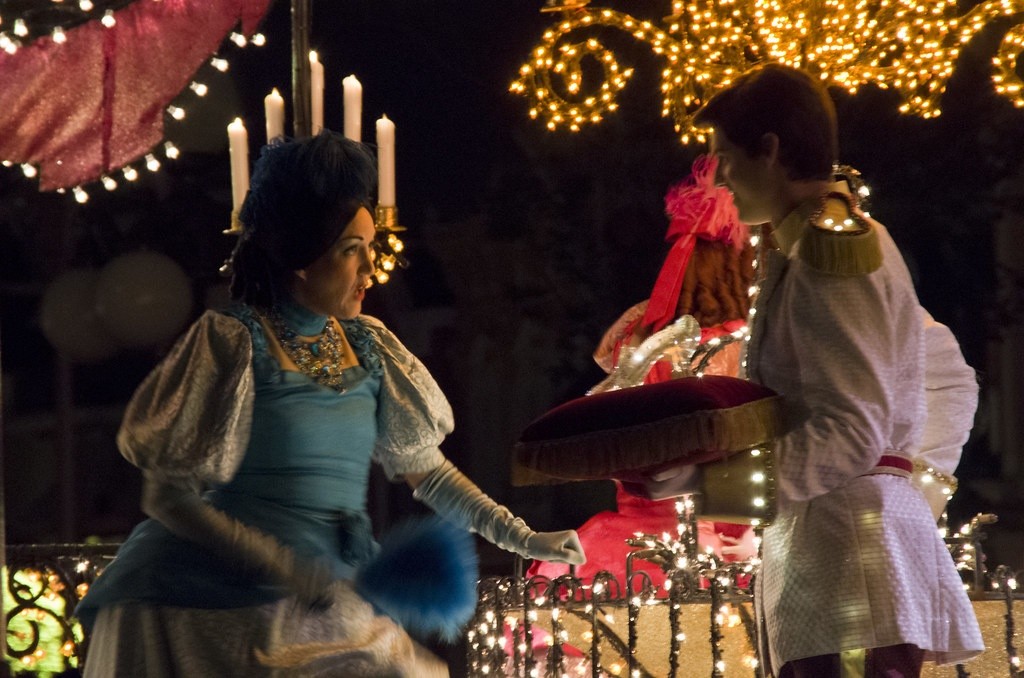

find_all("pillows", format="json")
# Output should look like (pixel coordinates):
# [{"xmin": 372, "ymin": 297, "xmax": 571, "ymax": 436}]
[{"xmin": 507, "ymin": 373, "xmax": 811, "ymax": 491}]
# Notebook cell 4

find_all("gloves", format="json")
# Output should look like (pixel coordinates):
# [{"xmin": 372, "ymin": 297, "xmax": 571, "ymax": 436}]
[
  {"xmin": 413, "ymin": 459, "xmax": 587, "ymax": 565},
  {"xmin": 140, "ymin": 478, "xmax": 335, "ymax": 609},
  {"xmin": 645, "ymin": 465, "xmax": 704, "ymax": 501}
]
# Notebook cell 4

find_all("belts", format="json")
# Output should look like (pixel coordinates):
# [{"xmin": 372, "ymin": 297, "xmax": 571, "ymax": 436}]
[{"xmin": 865, "ymin": 448, "xmax": 915, "ymax": 479}]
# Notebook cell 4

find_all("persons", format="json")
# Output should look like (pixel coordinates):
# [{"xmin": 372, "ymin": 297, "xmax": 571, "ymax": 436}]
[
  {"xmin": 75, "ymin": 131, "xmax": 588, "ymax": 678},
  {"xmin": 621, "ymin": 64, "xmax": 986, "ymax": 678}
]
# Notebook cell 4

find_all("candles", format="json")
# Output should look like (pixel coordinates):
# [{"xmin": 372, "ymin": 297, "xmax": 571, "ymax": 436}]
[
  {"xmin": 263, "ymin": 87, "xmax": 285, "ymax": 143},
  {"xmin": 227, "ymin": 116, "xmax": 252, "ymax": 228},
  {"xmin": 375, "ymin": 115, "xmax": 396, "ymax": 206},
  {"xmin": 308, "ymin": 50, "xmax": 325, "ymax": 136},
  {"xmin": 343, "ymin": 73, "xmax": 363, "ymax": 143}
]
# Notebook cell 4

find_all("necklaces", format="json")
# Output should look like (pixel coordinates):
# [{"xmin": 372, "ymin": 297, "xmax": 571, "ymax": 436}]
[{"xmin": 265, "ymin": 305, "xmax": 346, "ymax": 395}]
